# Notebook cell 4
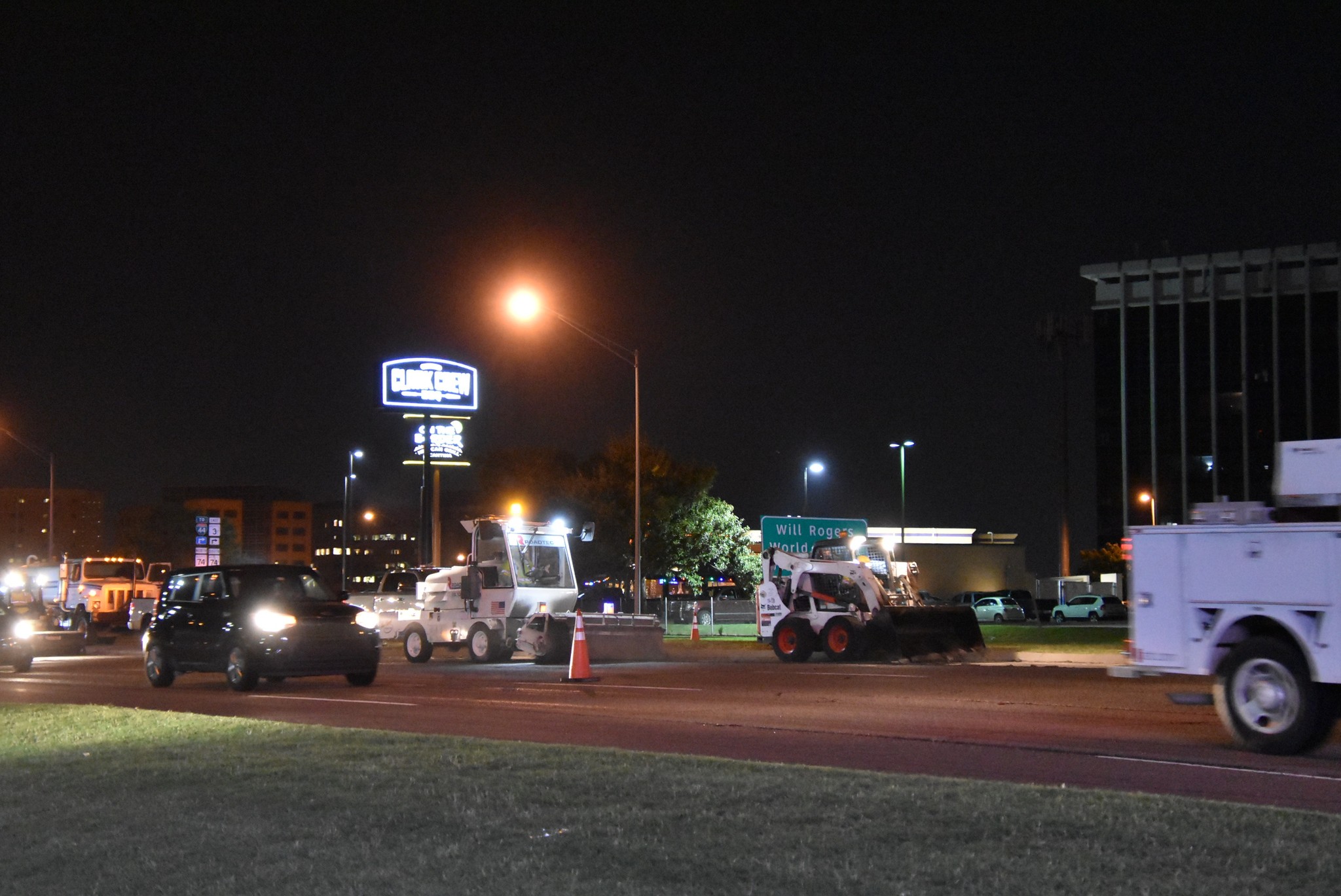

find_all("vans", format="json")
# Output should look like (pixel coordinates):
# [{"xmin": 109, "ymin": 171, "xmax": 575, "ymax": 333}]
[{"xmin": 997, "ymin": 590, "xmax": 1033, "ymax": 619}]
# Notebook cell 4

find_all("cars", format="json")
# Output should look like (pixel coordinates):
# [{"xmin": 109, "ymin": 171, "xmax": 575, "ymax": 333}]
[
  {"xmin": 971, "ymin": 594, "xmax": 1025, "ymax": 623},
  {"xmin": 952, "ymin": 592, "xmax": 990, "ymax": 605},
  {"xmin": 0, "ymin": 601, "xmax": 34, "ymax": 672}
]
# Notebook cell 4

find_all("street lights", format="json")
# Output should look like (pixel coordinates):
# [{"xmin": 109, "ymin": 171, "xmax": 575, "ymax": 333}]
[
  {"xmin": 890, "ymin": 439, "xmax": 914, "ymax": 543},
  {"xmin": 348, "ymin": 451, "xmax": 363, "ymax": 587},
  {"xmin": 1140, "ymin": 491, "xmax": 1155, "ymax": 526},
  {"xmin": 803, "ymin": 463, "xmax": 823, "ymax": 516},
  {"xmin": 342, "ymin": 474, "xmax": 356, "ymax": 591},
  {"xmin": 512, "ymin": 291, "xmax": 641, "ymax": 614}
]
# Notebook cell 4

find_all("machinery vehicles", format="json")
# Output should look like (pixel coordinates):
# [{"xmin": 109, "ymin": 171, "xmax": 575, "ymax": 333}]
[
  {"xmin": 1121, "ymin": 439, "xmax": 1341, "ymax": 753},
  {"xmin": 759, "ymin": 531, "xmax": 986, "ymax": 663},
  {"xmin": 701, "ymin": 576, "xmax": 740, "ymax": 599},
  {"xmin": 378, "ymin": 503, "xmax": 668, "ymax": 664},
  {"xmin": 18, "ymin": 551, "xmax": 171, "ymax": 643},
  {"xmin": 0, "ymin": 569, "xmax": 88, "ymax": 655}
]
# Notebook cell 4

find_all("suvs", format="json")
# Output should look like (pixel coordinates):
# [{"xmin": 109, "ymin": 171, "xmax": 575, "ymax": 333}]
[
  {"xmin": 1052, "ymin": 595, "xmax": 1126, "ymax": 623},
  {"xmin": 141, "ymin": 565, "xmax": 379, "ymax": 692}
]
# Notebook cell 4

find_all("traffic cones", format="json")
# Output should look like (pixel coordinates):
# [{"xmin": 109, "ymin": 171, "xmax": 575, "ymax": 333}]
[
  {"xmin": 560, "ymin": 608, "xmax": 601, "ymax": 683},
  {"xmin": 690, "ymin": 613, "xmax": 700, "ymax": 640}
]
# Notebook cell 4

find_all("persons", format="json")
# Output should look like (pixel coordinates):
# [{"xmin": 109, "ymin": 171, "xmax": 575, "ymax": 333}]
[{"xmin": 501, "ymin": 546, "xmax": 550, "ymax": 586}]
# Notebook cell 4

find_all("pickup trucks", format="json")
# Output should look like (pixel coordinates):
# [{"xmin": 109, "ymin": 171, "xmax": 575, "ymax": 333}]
[
  {"xmin": 670, "ymin": 585, "xmax": 758, "ymax": 626},
  {"xmin": 572, "ymin": 587, "xmax": 662, "ymax": 619}
]
